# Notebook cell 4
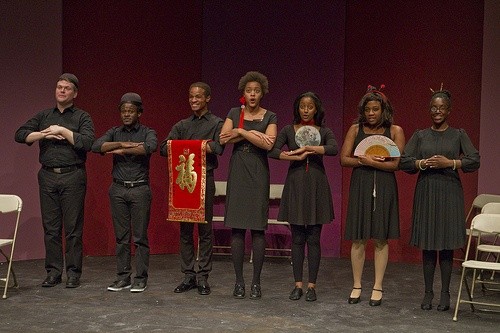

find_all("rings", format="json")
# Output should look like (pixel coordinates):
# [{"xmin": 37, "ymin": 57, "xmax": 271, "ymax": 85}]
[{"xmin": 291, "ymin": 151, "xmax": 292, "ymax": 152}]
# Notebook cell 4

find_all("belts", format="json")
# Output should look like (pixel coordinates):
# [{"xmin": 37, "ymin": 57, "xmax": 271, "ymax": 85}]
[
  {"xmin": 112, "ymin": 178, "xmax": 148, "ymax": 188},
  {"xmin": 42, "ymin": 165, "xmax": 71, "ymax": 174},
  {"xmin": 234, "ymin": 143, "xmax": 262, "ymax": 154}
]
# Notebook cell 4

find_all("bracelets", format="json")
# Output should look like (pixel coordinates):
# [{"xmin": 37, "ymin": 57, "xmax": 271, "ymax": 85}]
[
  {"xmin": 452, "ymin": 160, "xmax": 456, "ymax": 170},
  {"xmin": 419, "ymin": 160, "xmax": 427, "ymax": 170}
]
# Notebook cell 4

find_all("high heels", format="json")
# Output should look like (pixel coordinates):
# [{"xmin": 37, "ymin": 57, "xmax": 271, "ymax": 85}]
[
  {"xmin": 421, "ymin": 291, "xmax": 434, "ymax": 310},
  {"xmin": 349, "ymin": 288, "xmax": 363, "ymax": 304},
  {"xmin": 369, "ymin": 288, "xmax": 384, "ymax": 306},
  {"xmin": 437, "ymin": 290, "xmax": 450, "ymax": 311}
]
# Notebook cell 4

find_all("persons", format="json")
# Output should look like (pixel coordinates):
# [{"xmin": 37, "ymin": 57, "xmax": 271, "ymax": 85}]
[
  {"xmin": 160, "ymin": 81, "xmax": 225, "ymax": 295},
  {"xmin": 14, "ymin": 73, "xmax": 94, "ymax": 288},
  {"xmin": 268, "ymin": 90, "xmax": 338, "ymax": 302},
  {"xmin": 219, "ymin": 72, "xmax": 278, "ymax": 299},
  {"xmin": 90, "ymin": 93, "xmax": 159, "ymax": 292},
  {"xmin": 340, "ymin": 85, "xmax": 406, "ymax": 307},
  {"xmin": 400, "ymin": 89, "xmax": 480, "ymax": 311}
]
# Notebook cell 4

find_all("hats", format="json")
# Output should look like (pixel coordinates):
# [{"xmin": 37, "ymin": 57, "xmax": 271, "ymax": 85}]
[
  {"xmin": 59, "ymin": 73, "xmax": 79, "ymax": 88},
  {"xmin": 120, "ymin": 93, "xmax": 142, "ymax": 105}
]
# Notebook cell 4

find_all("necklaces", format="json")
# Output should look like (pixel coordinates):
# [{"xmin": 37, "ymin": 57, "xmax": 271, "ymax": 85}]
[{"xmin": 431, "ymin": 125, "xmax": 449, "ymax": 132}]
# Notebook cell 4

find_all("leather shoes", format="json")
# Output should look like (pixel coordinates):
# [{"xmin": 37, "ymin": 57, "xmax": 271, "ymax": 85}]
[
  {"xmin": 197, "ymin": 281, "xmax": 210, "ymax": 295},
  {"xmin": 249, "ymin": 284, "xmax": 262, "ymax": 299},
  {"xmin": 66, "ymin": 278, "xmax": 80, "ymax": 288},
  {"xmin": 233, "ymin": 281, "xmax": 245, "ymax": 299},
  {"xmin": 107, "ymin": 279, "xmax": 131, "ymax": 291},
  {"xmin": 174, "ymin": 281, "xmax": 197, "ymax": 293},
  {"xmin": 130, "ymin": 283, "xmax": 148, "ymax": 291},
  {"xmin": 289, "ymin": 288, "xmax": 303, "ymax": 300},
  {"xmin": 305, "ymin": 287, "xmax": 316, "ymax": 301},
  {"xmin": 42, "ymin": 274, "xmax": 62, "ymax": 286}
]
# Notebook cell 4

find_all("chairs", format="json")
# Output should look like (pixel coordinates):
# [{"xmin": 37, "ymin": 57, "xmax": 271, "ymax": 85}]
[
  {"xmin": 249, "ymin": 184, "xmax": 293, "ymax": 266},
  {"xmin": 448, "ymin": 193, "xmax": 500, "ymax": 321},
  {"xmin": 0, "ymin": 194, "xmax": 23, "ymax": 299},
  {"xmin": 194, "ymin": 180, "xmax": 232, "ymax": 260}
]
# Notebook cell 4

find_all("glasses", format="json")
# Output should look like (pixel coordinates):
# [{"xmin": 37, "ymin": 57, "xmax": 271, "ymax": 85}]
[{"xmin": 430, "ymin": 105, "xmax": 449, "ymax": 112}]
